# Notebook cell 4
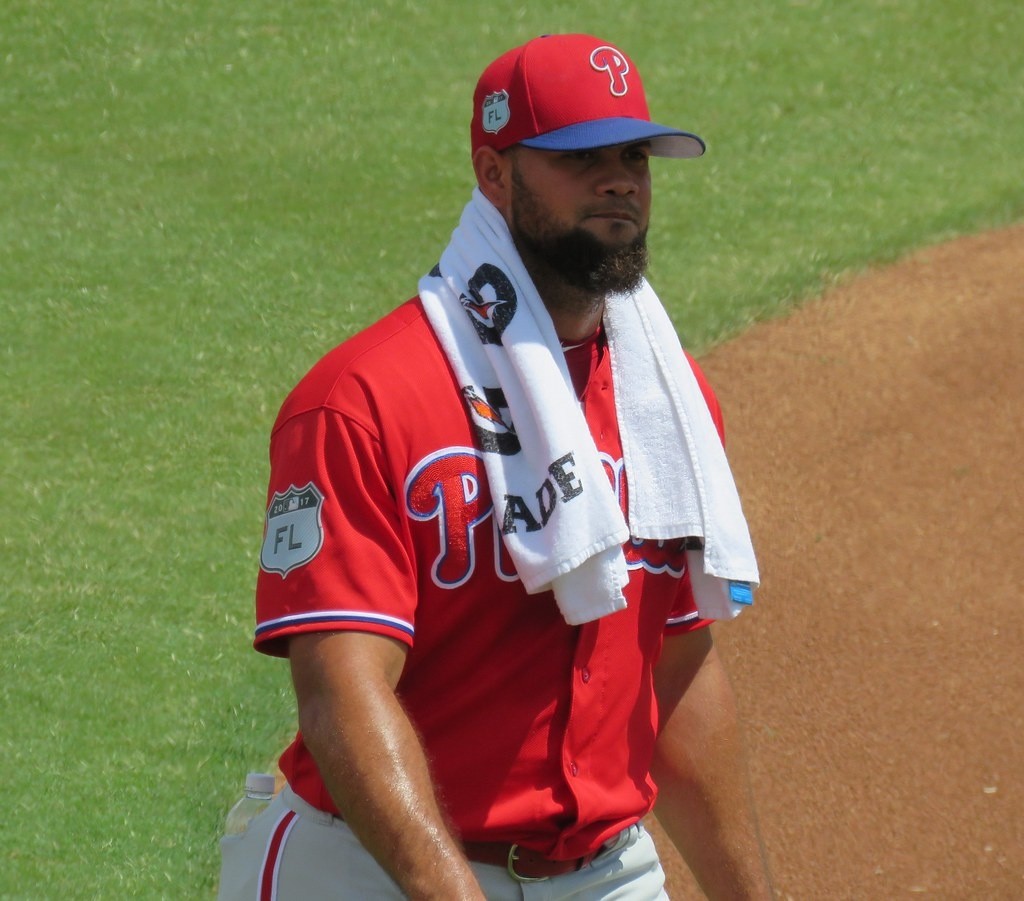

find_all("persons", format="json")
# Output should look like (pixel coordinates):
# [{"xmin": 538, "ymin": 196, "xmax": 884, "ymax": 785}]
[{"xmin": 220, "ymin": 33, "xmax": 774, "ymax": 900}]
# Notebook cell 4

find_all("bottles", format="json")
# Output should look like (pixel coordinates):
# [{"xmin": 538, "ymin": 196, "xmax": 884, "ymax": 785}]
[{"xmin": 224, "ymin": 774, "xmax": 275, "ymax": 837}]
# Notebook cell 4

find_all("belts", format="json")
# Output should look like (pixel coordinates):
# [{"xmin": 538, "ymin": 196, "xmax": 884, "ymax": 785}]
[{"xmin": 332, "ymin": 813, "xmax": 606, "ymax": 877}]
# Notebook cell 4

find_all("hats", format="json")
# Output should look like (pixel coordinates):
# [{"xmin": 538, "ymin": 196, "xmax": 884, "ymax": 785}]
[{"xmin": 470, "ymin": 33, "xmax": 706, "ymax": 160}]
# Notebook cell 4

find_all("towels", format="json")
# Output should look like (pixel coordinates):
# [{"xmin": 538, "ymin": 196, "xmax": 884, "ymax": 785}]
[{"xmin": 417, "ymin": 180, "xmax": 762, "ymax": 627}]
